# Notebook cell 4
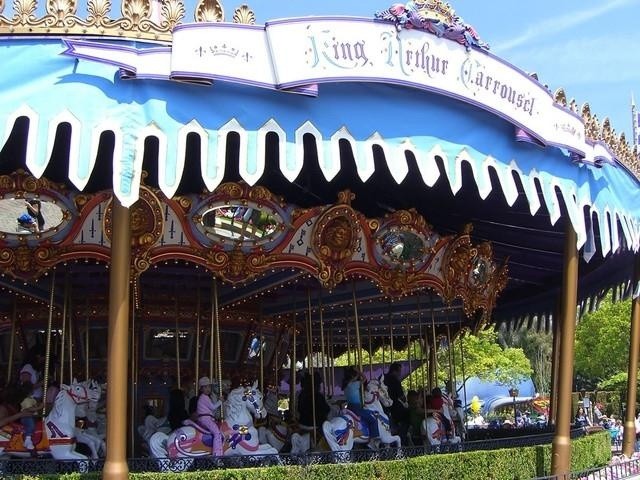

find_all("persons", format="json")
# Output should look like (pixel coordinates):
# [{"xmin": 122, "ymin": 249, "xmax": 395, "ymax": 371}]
[
  {"xmin": 344, "ymin": 370, "xmax": 379, "ymax": 450},
  {"xmin": 407, "ymin": 387, "xmax": 452, "ymax": 446},
  {"xmin": 378, "ymin": 363, "xmax": 410, "ymax": 445},
  {"xmin": 197, "ymin": 376, "xmax": 225, "ymax": 467},
  {"xmin": 466, "ymin": 403, "xmax": 640, "ymax": 452},
  {"xmin": 0, "ymin": 388, "xmax": 44, "ymax": 450}
]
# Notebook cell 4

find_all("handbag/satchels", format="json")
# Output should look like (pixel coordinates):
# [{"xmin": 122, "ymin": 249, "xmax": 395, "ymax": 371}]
[{"xmin": 400, "ymin": 400, "xmax": 409, "ymax": 408}]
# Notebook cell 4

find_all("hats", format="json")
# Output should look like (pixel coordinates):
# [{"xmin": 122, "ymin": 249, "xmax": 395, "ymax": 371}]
[
  {"xmin": 199, "ymin": 376, "xmax": 214, "ymax": 389},
  {"xmin": 525, "ymin": 411, "xmax": 531, "ymax": 414},
  {"xmin": 538, "ymin": 415, "xmax": 545, "ymax": 420}
]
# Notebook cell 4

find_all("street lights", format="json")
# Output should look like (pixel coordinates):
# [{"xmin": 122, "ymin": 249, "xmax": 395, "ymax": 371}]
[{"xmin": 510, "ymin": 387, "xmax": 519, "ymax": 429}]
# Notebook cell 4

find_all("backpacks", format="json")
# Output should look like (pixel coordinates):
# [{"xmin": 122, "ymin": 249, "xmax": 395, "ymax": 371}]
[{"xmin": 46, "ymin": 386, "xmax": 59, "ymax": 404}]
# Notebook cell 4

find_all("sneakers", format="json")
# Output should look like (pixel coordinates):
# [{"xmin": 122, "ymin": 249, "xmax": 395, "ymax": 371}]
[
  {"xmin": 368, "ymin": 442, "xmax": 377, "ymax": 449},
  {"xmin": 213, "ymin": 459, "xmax": 224, "ymax": 466},
  {"xmin": 448, "ymin": 438, "xmax": 453, "ymax": 442},
  {"xmin": 23, "ymin": 440, "xmax": 34, "ymax": 449}
]
